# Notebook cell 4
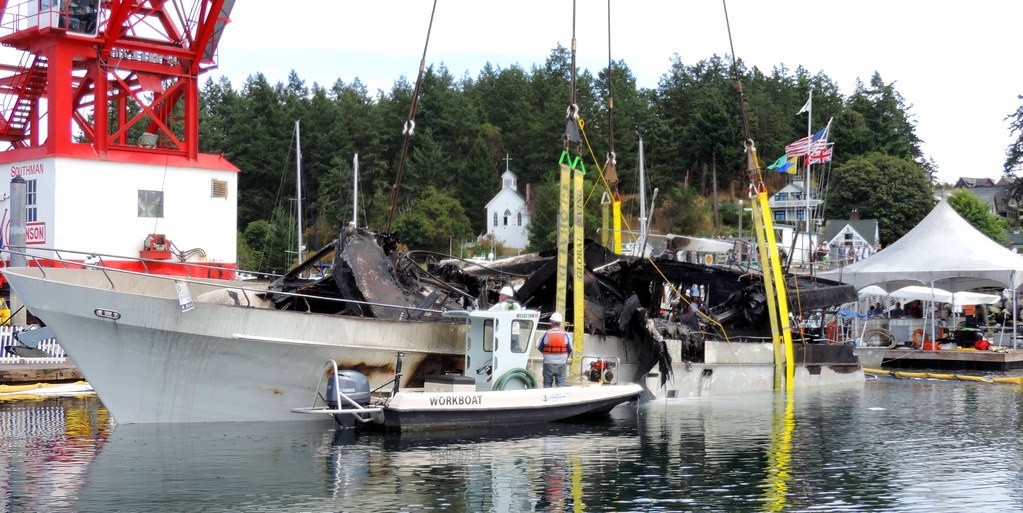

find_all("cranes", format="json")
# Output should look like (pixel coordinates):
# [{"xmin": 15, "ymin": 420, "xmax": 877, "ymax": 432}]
[{"xmin": 0, "ymin": 0, "xmax": 242, "ymax": 288}]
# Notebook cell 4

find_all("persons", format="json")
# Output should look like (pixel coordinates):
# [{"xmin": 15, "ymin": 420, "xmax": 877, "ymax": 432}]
[
  {"xmin": 812, "ymin": 241, "xmax": 890, "ymax": 265},
  {"xmin": 488, "ymin": 285, "xmax": 523, "ymax": 352},
  {"xmin": 535, "ymin": 313, "xmax": 572, "ymax": 387},
  {"xmin": 867, "ymin": 299, "xmax": 903, "ymax": 318}
]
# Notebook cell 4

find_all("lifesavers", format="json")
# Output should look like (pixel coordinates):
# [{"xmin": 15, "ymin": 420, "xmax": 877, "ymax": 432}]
[{"xmin": 913, "ymin": 328, "xmax": 930, "ymax": 349}]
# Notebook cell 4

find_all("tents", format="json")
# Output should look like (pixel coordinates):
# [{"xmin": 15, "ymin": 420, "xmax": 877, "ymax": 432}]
[{"xmin": 821, "ymin": 196, "xmax": 1023, "ymax": 307}]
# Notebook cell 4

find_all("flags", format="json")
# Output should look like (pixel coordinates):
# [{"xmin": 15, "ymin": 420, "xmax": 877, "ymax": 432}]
[
  {"xmin": 765, "ymin": 127, "xmax": 832, "ymax": 174},
  {"xmin": 795, "ymin": 98, "xmax": 811, "ymax": 115}
]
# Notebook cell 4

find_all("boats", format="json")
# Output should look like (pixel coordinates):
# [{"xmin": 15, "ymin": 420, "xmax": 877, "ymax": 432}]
[
  {"xmin": 289, "ymin": 308, "xmax": 647, "ymax": 434},
  {"xmin": 2, "ymin": 228, "xmax": 865, "ymax": 426}
]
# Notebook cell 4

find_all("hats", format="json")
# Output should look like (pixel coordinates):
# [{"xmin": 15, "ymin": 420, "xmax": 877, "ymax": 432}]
[
  {"xmin": 498, "ymin": 286, "xmax": 514, "ymax": 298},
  {"xmin": 549, "ymin": 313, "xmax": 563, "ymax": 322},
  {"xmin": 691, "ymin": 287, "xmax": 700, "ymax": 297}
]
